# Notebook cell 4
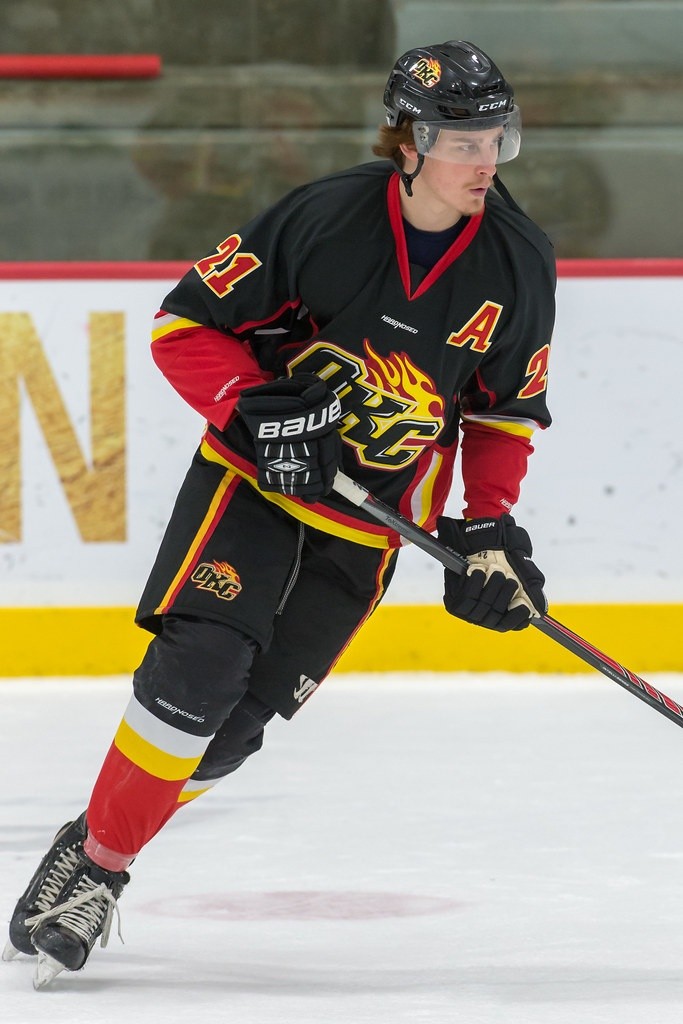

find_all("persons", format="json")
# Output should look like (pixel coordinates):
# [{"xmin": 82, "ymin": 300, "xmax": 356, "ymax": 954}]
[{"xmin": 2, "ymin": 42, "xmax": 556, "ymax": 990}]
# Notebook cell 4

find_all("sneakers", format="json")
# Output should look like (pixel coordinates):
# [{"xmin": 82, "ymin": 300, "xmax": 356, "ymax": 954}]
[
  {"xmin": 2, "ymin": 808, "xmax": 89, "ymax": 964},
  {"xmin": 24, "ymin": 846, "xmax": 130, "ymax": 989}
]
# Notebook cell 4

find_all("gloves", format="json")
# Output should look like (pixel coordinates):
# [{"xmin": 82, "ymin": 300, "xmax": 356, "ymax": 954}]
[
  {"xmin": 236, "ymin": 374, "xmax": 344, "ymax": 504},
  {"xmin": 435, "ymin": 515, "xmax": 548, "ymax": 633}
]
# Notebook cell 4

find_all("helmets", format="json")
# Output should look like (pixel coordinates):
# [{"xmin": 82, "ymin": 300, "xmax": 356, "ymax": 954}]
[{"xmin": 382, "ymin": 39, "xmax": 515, "ymax": 131}]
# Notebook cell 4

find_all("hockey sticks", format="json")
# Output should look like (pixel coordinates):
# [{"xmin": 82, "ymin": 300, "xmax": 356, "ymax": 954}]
[{"xmin": 332, "ymin": 469, "xmax": 683, "ymax": 728}]
